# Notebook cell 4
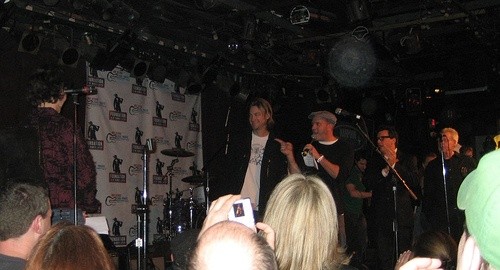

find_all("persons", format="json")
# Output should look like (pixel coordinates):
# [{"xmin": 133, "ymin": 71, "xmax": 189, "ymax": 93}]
[
  {"xmin": 362, "ymin": 125, "xmax": 420, "ymax": 270},
  {"xmin": 5, "ymin": 70, "xmax": 101, "ymax": 225},
  {"xmin": 23, "ymin": 222, "xmax": 115, "ymax": 270},
  {"xmin": 274, "ymin": 111, "xmax": 353, "ymax": 246},
  {"xmin": 0, "ymin": 178, "xmax": 53, "ymax": 270},
  {"xmin": 212, "ymin": 97, "xmax": 289, "ymax": 223},
  {"xmin": 168, "ymin": 174, "xmax": 356, "ymax": 270},
  {"xmin": 423, "ymin": 128, "xmax": 477, "ymax": 247},
  {"xmin": 342, "ymin": 133, "xmax": 500, "ymax": 270},
  {"xmin": 235, "ymin": 205, "xmax": 242, "ymax": 217}
]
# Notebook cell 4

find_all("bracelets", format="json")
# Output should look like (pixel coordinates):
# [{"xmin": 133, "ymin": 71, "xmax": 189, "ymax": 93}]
[{"xmin": 316, "ymin": 154, "xmax": 324, "ymax": 163}]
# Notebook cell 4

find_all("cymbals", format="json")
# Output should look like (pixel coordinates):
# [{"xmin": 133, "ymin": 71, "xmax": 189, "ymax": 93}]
[
  {"xmin": 182, "ymin": 175, "xmax": 217, "ymax": 182},
  {"xmin": 161, "ymin": 147, "xmax": 194, "ymax": 157}
]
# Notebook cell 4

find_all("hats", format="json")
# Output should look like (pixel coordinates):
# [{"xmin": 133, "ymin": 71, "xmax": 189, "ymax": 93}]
[
  {"xmin": 456, "ymin": 148, "xmax": 500, "ymax": 270},
  {"xmin": 308, "ymin": 111, "xmax": 337, "ymax": 124}
]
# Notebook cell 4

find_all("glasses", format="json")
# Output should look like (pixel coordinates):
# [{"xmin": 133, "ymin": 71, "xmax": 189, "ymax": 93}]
[{"xmin": 375, "ymin": 136, "xmax": 394, "ymax": 141}]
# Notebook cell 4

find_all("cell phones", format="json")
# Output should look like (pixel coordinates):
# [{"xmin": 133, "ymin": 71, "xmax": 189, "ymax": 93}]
[{"xmin": 229, "ymin": 198, "xmax": 257, "ymax": 233}]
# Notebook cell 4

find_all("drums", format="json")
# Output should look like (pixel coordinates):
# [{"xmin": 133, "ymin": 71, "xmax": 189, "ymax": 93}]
[
  {"xmin": 164, "ymin": 200, "xmax": 202, "ymax": 231},
  {"xmin": 189, "ymin": 184, "xmax": 207, "ymax": 205}
]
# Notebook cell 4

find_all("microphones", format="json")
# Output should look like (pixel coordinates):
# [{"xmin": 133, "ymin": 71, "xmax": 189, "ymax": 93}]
[
  {"xmin": 335, "ymin": 108, "xmax": 361, "ymax": 120},
  {"xmin": 430, "ymin": 131, "xmax": 446, "ymax": 137},
  {"xmin": 303, "ymin": 135, "xmax": 316, "ymax": 156},
  {"xmin": 64, "ymin": 85, "xmax": 97, "ymax": 95}
]
